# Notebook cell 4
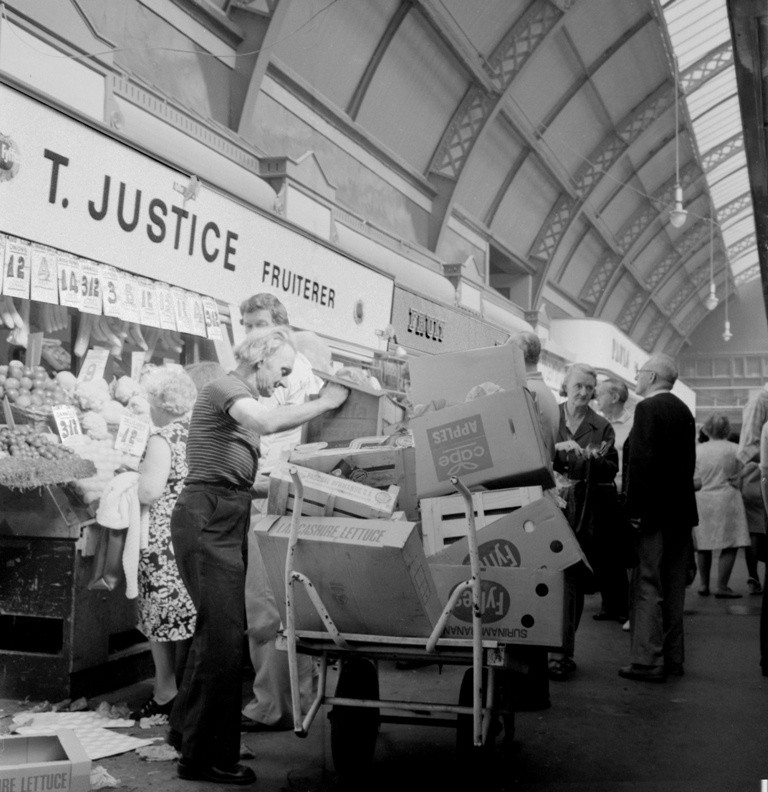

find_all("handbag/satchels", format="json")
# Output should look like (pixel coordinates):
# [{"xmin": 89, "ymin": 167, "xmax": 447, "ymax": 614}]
[{"xmin": 87, "ymin": 527, "xmax": 125, "ymax": 593}]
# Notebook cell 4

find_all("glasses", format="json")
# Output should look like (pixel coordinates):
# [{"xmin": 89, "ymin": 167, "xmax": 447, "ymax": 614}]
[{"xmin": 635, "ymin": 368, "xmax": 654, "ymax": 376}]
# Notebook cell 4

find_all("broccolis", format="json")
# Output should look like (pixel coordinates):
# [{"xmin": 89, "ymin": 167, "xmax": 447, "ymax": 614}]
[{"xmin": 76, "ymin": 376, "xmax": 140, "ymax": 444}]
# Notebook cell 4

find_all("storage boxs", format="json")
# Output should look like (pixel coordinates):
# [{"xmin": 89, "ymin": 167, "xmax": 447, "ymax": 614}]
[{"xmin": 251, "ymin": 339, "xmax": 592, "ymax": 647}]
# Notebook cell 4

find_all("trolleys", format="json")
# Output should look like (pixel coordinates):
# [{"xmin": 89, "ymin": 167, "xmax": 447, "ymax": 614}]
[{"xmin": 284, "ymin": 465, "xmax": 563, "ymax": 781}]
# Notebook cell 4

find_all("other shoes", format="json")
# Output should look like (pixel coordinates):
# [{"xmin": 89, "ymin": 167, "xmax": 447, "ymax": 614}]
[
  {"xmin": 622, "ymin": 620, "xmax": 630, "ymax": 630},
  {"xmin": 715, "ymin": 586, "xmax": 743, "ymax": 599},
  {"xmin": 698, "ymin": 586, "xmax": 710, "ymax": 596},
  {"xmin": 239, "ymin": 712, "xmax": 294, "ymax": 732},
  {"xmin": 176, "ymin": 755, "xmax": 256, "ymax": 785},
  {"xmin": 167, "ymin": 728, "xmax": 193, "ymax": 750},
  {"xmin": 592, "ymin": 611, "xmax": 623, "ymax": 621},
  {"xmin": 548, "ymin": 657, "xmax": 568, "ymax": 683},
  {"xmin": 618, "ymin": 667, "xmax": 665, "ymax": 683},
  {"xmin": 130, "ymin": 693, "xmax": 178, "ymax": 722},
  {"xmin": 663, "ymin": 665, "xmax": 685, "ymax": 677},
  {"xmin": 746, "ymin": 577, "xmax": 762, "ymax": 594}
]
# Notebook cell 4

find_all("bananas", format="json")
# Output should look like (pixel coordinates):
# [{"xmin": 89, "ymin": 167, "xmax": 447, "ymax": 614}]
[{"xmin": 0, "ymin": 295, "xmax": 186, "ymax": 354}]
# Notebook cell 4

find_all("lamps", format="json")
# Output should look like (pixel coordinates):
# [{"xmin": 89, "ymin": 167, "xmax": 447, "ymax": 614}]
[
  {"xmin": 669, "ymin": 53, "xmax": 688, "ymax": 229},
  {"xmin": 704, "ymin": 188, "xmax": 718, "ymax": 310},
  {"xmin": 722, "ymin": 257, "xmax": 734, "ymax": 343}
]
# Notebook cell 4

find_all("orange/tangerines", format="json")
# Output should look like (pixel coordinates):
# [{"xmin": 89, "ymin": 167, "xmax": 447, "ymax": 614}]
[{"xmin": 0, "ymin": 359, "xmax": 66, "ymax": 414}]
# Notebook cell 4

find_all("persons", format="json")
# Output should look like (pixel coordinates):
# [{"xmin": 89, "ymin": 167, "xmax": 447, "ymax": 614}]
[
  {"xmin": 545, "ymin": 354, "xmax": 767, "ymax": 683},
  {"xmin": 501, "ymin": 332, "xmax": 561, "ymax": 475},
  {"xmin": 128, "ymin": 288, "xmax": 335, "ymax": 734},
  {"xmin": 168, "ymin": 325, "xmax": 351, "ymax": 784},
  {"xmin": 694, "ymin": 412, "xmax": 752, "ymax": 599}
]
